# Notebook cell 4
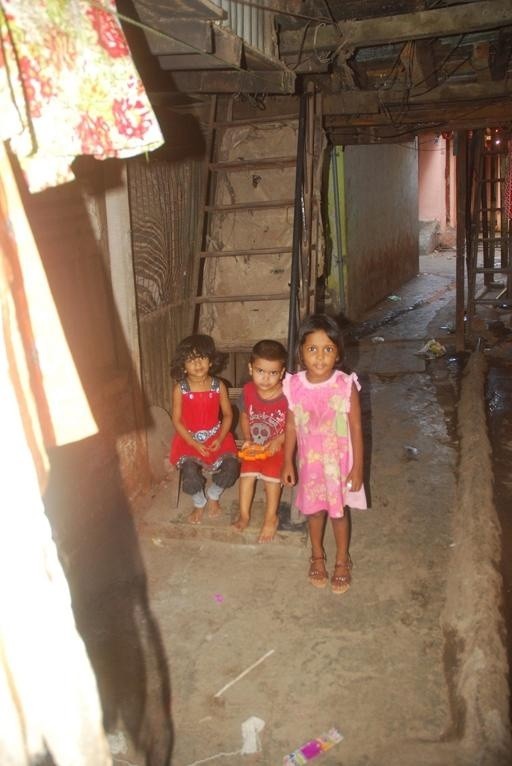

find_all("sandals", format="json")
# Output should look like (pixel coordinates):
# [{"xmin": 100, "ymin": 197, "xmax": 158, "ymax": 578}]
[
  {"xmin": 308, "ymin": 552, "xmax": 329, "ymax": 588},
  {"xmin": 331, "ymin": 553, "xmax": 353, "ymax": 595}
]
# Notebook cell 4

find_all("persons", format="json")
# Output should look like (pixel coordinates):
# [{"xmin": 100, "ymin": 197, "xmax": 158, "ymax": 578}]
[
  {"xmin": 280, "ymin": 314, "xmax": 368, "ymax": 593},
  {"xmin": 170, "ymin": 335, "xmax": 240, "ymax": 524},
  {"xmin": 234, "ymin": 340, "xmax": 288, "ymax": 543}
]
